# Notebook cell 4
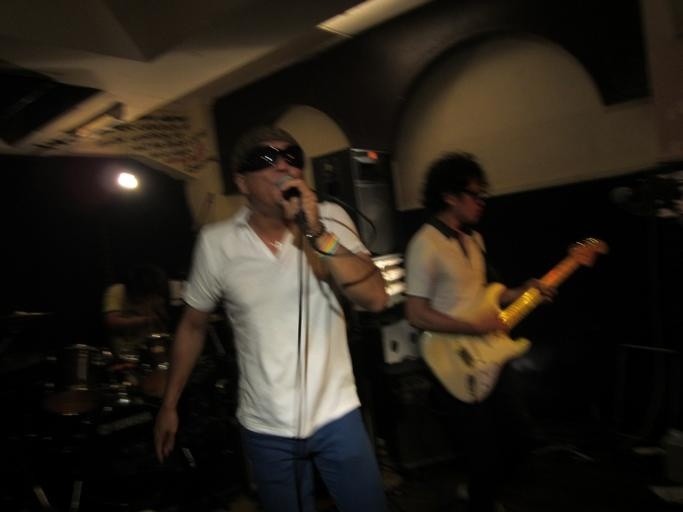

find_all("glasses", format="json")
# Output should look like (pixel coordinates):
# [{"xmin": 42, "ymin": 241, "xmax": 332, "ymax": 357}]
[
  {"xmin": 235, "ymin": 141, "xmax": 304, "ymax": 176},
  {"xmin": 459, "ymin": 185, "xmax": 489, "ymax": 202}
]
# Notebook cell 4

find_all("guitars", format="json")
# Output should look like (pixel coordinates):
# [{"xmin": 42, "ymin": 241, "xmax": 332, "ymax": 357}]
[{"xmin": 421, "ymin": 238, "xmax": 607, "ymax": 404}]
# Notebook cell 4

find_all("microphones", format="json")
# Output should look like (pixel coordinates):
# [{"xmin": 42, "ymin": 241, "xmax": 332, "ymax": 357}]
[{"xmin": 280, "ymin": 174, "xmax": 298, "ymax": 219}]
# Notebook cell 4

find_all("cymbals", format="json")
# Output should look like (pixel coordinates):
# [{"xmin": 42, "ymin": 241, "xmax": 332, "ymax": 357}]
[{"xmin": 0, "ymin": 311, "xmax": 51, "ymax": 317}]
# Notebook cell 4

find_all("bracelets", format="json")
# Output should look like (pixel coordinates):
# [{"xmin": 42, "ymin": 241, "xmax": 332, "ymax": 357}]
[{"xmin": 313, "ymin": 233, "xmax": 340, "ymax": 259}]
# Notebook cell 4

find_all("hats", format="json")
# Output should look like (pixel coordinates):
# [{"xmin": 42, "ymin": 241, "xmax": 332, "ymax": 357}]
[{"xmin": 227, "ymin": 124, "xmax": 304, "ymax": 178}]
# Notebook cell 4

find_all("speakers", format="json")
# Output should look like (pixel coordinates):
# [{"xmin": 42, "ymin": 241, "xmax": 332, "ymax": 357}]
[{"xmin": 310, "ymin": 147, "xmax": 396, "ymax": 255}]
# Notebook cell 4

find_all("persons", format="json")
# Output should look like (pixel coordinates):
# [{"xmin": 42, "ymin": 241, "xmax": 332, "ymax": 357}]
[
  {"xmin": 404, "ymin": 150, "xmax": 557, "ymax": 512},
  {"xmin": 152, "ymin": 123, "xmax": 389, "ymax": 512},
  {"xmin": 103, "ymin": 264, "xmax": 164, "ymax": 334}
]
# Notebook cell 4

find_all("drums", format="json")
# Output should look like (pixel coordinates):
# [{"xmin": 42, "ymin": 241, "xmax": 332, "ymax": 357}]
[
  {"xmin": 134, "ymin": 333, "xmax": 172, "ymax": 399},
  {"xmin": 102, "ymin": 349, "xmax": 138, "ymax": 412},
  {"xmin": 43, "ymin": 344, "xmax": 106, "ymax": 414}
]
